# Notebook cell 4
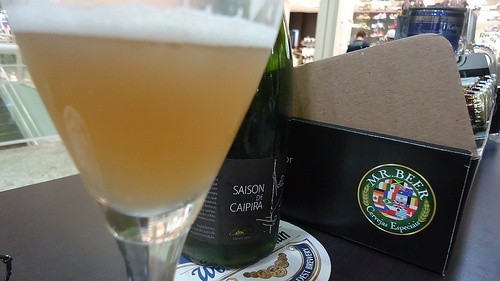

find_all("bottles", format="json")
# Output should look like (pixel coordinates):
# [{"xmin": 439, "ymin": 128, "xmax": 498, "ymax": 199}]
[{"xmin": 181, "ymin": 19, "xmax": 293, "ymax": 267}]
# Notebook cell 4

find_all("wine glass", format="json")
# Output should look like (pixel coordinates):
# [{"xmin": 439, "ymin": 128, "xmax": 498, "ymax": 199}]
[{"xmin": 1, "ymin": 1, "xmax": 283, "ymax": 280}]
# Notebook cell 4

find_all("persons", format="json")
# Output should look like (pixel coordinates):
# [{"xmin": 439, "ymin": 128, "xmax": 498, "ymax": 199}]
[
  {"xmin": 346, "ymin": 31, "xmax": 370, "ymax": 54},
  {"xmin": 379, "ymin": 28, "xmax": 396, "ymax": 43}
]
